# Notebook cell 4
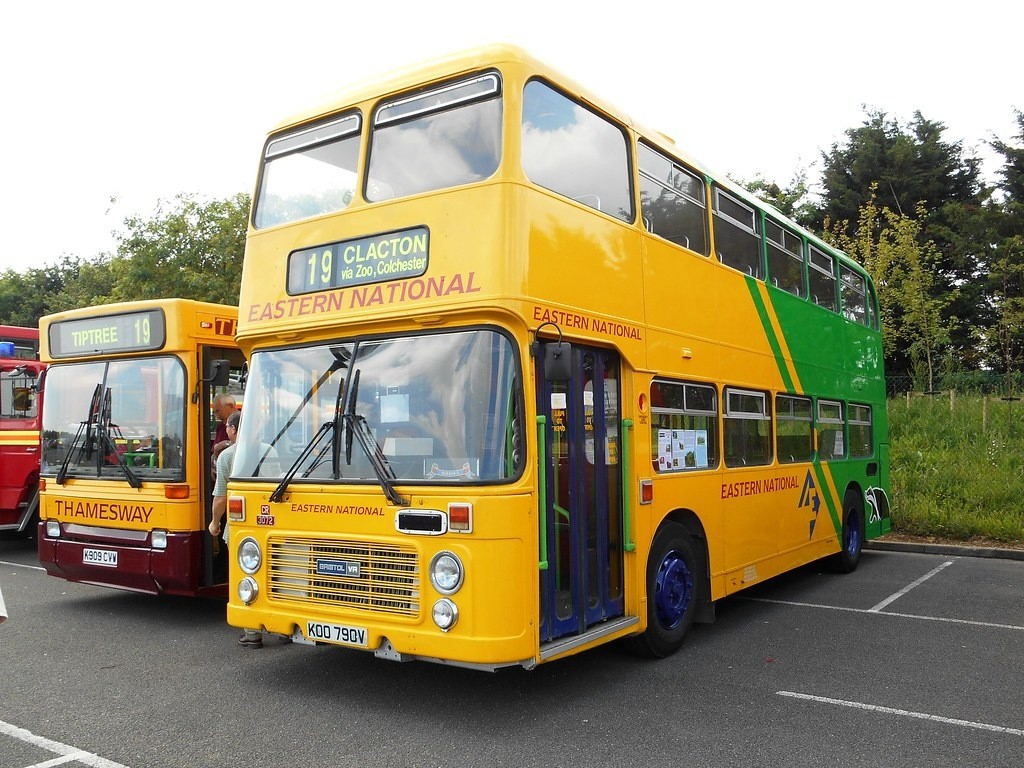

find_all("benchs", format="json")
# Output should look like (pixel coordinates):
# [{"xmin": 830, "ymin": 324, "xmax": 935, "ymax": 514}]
[{"xmin": 707, "ymin": 451, "xmax": 833, "ymax": 467}]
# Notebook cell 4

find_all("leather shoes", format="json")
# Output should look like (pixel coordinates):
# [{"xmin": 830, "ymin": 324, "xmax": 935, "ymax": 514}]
[{"xmin": 238, "ymin": 634, "xmax": 264, "ymax": 650}]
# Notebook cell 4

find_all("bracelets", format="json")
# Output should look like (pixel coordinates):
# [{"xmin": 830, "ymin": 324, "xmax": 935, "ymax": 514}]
[{"xmin": 226, "ymin": 440, "xmax": 230, "ymax": 447}]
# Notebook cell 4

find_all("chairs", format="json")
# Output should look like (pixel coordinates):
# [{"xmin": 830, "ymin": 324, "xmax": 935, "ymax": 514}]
[{"xmin": 574, "ymin": 193, "xmax": 850, "ymax": 318}]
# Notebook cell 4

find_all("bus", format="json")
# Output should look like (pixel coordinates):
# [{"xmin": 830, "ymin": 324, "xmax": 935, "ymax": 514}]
[
  {"xmin": 38, "ymin": 297, "xmax": 249, "ymax": 604},
  {"xmin": 226, "ymin": 47, "xmax": 897, "ymax": 675},
  {"xmin": 0, "ymin": 325, "xmax": 45, "ymax": 545}
]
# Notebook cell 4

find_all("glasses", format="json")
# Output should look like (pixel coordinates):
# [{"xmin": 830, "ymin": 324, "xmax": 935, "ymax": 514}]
[{"xmin": 215, "ymin": 404, "xmax": 228, "ymax": 414}]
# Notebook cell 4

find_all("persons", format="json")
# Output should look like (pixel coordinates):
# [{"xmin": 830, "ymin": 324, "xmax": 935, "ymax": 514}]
[
  {"xmin": 211, "ymin": 393, "xmax": 241, "ymax": 481},
  {"xmin": 136, "ymin": 427, "xmax": 151, "ymax": 451},
  {"xmin": 208, "ymin": 411, "xmax": 291, "ymax": 647}
]
